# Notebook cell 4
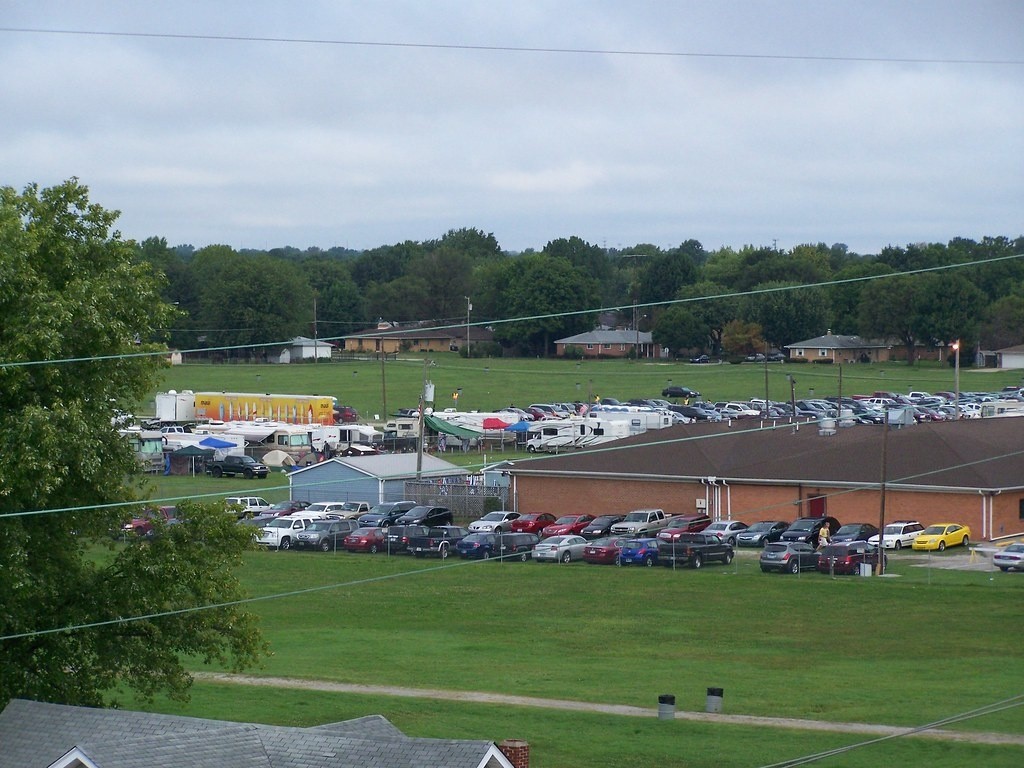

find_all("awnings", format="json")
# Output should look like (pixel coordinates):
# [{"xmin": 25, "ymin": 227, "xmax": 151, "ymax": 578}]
[{"xmin": 220, "ymin": 427, "xmax": 278, "ymax": 442}]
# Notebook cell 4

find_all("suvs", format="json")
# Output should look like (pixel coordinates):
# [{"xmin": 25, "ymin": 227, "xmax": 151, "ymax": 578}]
[
  {"xmin": 817, "ymin": 540, "xmax": 889, "ymax": 574},
  {"xmin": 394, "ymin": 505, "xmax": 454, "ymax": 531},
  {"xmin": 656, "ymin": 514, "xmax": 713, "ymax": 545},
  {"xmin": 355, "ymin": 499, "xmax": 418, "ymax": 528},
  {"xmin": 491, "ymin": 533, "xmax": 539, "ymax": 562},
  {"xmin": 759, "ymin": 539, "xmax": 823, "ymax": 573},
  {"xmin": 778, "ymin": 516, "xmax": 841, "ymax": 548}
]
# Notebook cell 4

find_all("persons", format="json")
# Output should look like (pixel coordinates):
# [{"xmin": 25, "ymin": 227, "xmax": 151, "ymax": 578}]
[
  {"xmin": 323, "ymin": 441, "xmax": 330, "ymax": 459},
  {"xmin": 509, "ymin": 403, "xmax": 516, "ymax": 408},
  {"xmin": 673, "ymin": 394, "xmax": 713, "ymax": 405},
  {"xmin": 573, "ymin": 405, "xmax": 583, "ymax": 416},
  {"xmin": 452, "ymin": 391, "xmax": 459, "ymax": 407},
  {"xmin": 594, "ymin": 393, "xmax": 600, "ymax": 402},
  {"xmin": 815, "ymin": 522, "xmax": 832, "ymax": 552}
]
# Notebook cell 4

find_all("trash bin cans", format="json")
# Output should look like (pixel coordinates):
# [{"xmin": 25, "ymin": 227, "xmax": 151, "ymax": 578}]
[
  {"xmin": 658, "ymin": 694, "xmax": 675, "ymax": 719},
  {"xmin": 706, "ymin": 687, "xmax": 724, "ymax": 712},
  {"xmin": 859, "ymin": 562, "xmax": 872, "ymax": 577}
]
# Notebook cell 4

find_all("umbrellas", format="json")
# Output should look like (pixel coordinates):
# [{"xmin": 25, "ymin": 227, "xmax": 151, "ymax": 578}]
[
  {"xmin": 172, "ymin": 444, "xmax": 212, "ymax": 478},
  {"xmin": 199, "ymin": 437, "xmax": 237, "ymax": 461}
]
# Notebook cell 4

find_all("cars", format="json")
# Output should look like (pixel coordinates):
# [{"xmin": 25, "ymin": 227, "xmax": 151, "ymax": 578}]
[
  {"xmin": 662, "ymin": 385, "xmax": 702, "ymax": 398},
  {"xmin": 579, "ymin": 514, "xmax": 627, "ymax": 541},
  {"xmin": 868, "ymin": 521, "xmax": 924, "ymax": 551},
  {"xmin": 456, "ymin": 533, "xmax": 500, "ymax": 558},
  {"xmin": 212, "ymin": 495, "xmax": 372, "ymax": 551},
  {"xmin": 511, "ymin": 511, "xmax": 557, "ymax": 538},
  {"xmin": 735, "ymin": 520, "xmax": 790, "ymax": 548},
  {"xmin": 697, "ymin": 521, "xmax": 749, "ymax": 548},
  {"xmin": 468, "ymin": 510, "xmax": 523, "ymax": 535},
  {"xmin": 619, "ymin": 538, "xmax": 671, "ymax": 567},
  {"xmin": 689, "ymin": 354, "xmax": 710, "ymax": 364},
  {"xmin": 767, "ymin": 353, "xmax": 785, "ymax": 361},
  {"xmin": 745, "ymin": 353, "xmax": 766, "ymax": 362},
  {"xmin": 381, "ymin": 525, "xmax": 429, "ymax": 556},
  {"xmin": 829, "ymin": 522, "xmax": 882, "ymax": 545},
  {"xmin": 343, "ymin": 527, "xmax": 384, "ymax": 554},
  {"xmin": 542, "ymin": 512, "xmax": 599, "ymax": 541},
  {"xmin": 582, "ymin": 537, "xmax": 626, "ymax": 567},
  {"xmin": 111, "ymin": 391, "xmax": 1023, "ymax": 479},
  {"xmin": 911, "ymin": 522, "xmax": 971, "ymax": 552},
  {"xmin": 992, "ymin": 542, "xmax": 1024, "ymax": 573},
  {"xmin": 531, "ymin": 534, "xmax": 589, "ymax": 563}
]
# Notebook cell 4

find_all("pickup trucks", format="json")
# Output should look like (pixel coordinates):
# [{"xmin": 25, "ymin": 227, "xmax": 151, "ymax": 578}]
[
  {"xmin": 656, "ymin": 533, "xmax": 736, "ymax": 569},
  {"xmin": 405, "ymin": 526, "xmax": 472, "ymax": 559},
  {"xmin": 610, "ymin": 508, "xmax": 683, "ymax": 539}
]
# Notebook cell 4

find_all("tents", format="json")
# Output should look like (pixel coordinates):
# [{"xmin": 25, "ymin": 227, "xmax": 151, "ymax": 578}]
[
  {"xmin": 261, "ymin": 450, "xmax": 327, "ymax": 468},
  {"xmin": 483, "ymin": 418, "xmax": 532, "ymax": 433}
]
